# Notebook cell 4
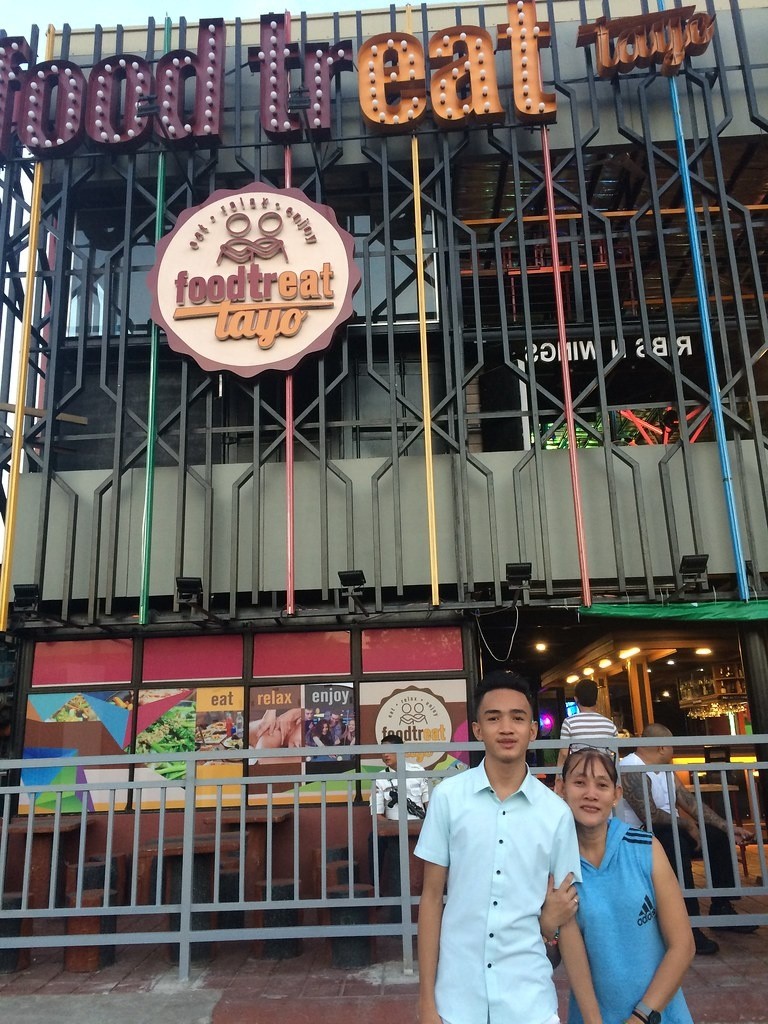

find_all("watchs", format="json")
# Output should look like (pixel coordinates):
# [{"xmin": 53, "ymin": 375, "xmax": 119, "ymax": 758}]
[{"xmin": 635, "ymin": 1000, "xmax": 662, "ymax": 1024}]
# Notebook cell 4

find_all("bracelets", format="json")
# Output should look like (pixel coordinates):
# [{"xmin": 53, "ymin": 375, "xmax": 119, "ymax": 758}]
[
  {"xmin": 632, "ymin": 1011, "xmax": 648, "ymax": 1024},
  {"xmin": 540, "ymin": 932, "xmax": 559, "ymax": 947}
]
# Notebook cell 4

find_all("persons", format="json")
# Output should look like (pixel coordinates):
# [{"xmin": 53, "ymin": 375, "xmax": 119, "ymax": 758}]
[
  {"xmin": 248, "ymin": 708, "xmax": 302, "ymax": 767},
  {"xmin": 304, "ymin": 705, "xmax": 357, "ymax": 760},
  {"xmin": 413, "ymin": 670, "xmax": 605, "ymax": 1024},
  {"xmin": 617, "ymin": 724, "xmax": 760, "ymax": 955},
  {"xmin": 538, "ymin": 742, "xmax": 697, "ymax": 1024},
  {"xmin": 554, "ymin": 678, "xmax": 622, "ymax": 794},
  {"xmin": 366, "ymin": 734, "xmax": 431, "ymax": 885}
]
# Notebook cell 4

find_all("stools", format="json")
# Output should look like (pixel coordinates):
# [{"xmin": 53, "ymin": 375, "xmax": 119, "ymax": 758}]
[
  {"xmin": 64, "ymin": 861, "xmax": 108, "ymax": 907},
  {"xmin": 219, "ymin": 868, "xmax": 246, "ymax": 929},
  {"xmin": 312, "ymin": 845, "xmax": 349, "ymax": 898},
  {"xmin": 89, "ymin": 852, "xmax": 127, "ymax": 907},
  {"xmin": 137, "ymin": 855, "xmax": 167, "ymax": 905},
  {"xmin": 0, "ymin": 892, "xmax": 35, "ymax": 972},
  {"xmin": 327, "ymin": 883, "xmax": 374, "ymax": 971},
  {"xmin": 64, "ymin": 889, "xmax": 120, "ymax": 975},
  {"xmin": 255, "ymin": 879, "xmax": 302, "ymax": 962},
  {"xmin": 318, "ymin": 859, "xmax": 359, "ymax": 899}
]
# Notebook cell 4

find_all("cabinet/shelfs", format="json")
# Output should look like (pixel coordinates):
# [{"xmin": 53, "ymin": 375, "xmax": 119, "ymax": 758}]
[{"xmin": 675, "ymin": 661, "xmax": 748, "ymax": 709}]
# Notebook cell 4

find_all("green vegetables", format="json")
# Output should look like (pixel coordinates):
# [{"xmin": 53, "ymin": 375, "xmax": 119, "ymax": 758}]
[{"xmin": 124, "ymin": 703, "xmax": 196, "ymax": 781}]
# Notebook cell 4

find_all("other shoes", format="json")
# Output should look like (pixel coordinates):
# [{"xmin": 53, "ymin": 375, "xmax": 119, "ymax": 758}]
[
  {"xmin": 707, "ymin": 905, "xmax": 759, "ymax": 934},
  {"xmin": 690, "ymin": 927, "xmax": 719, "ymax": 954}
]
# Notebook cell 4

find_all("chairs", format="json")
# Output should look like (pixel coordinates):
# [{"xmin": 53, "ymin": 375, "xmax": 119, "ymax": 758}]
[{"xmin": 704, "ymin": 744, "xmax": 750, "ymax": 878}]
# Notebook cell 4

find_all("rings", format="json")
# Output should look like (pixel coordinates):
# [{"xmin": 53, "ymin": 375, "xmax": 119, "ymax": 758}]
[{"xmin": 574, "ymin": 900, "xmax": 579, "ymax": 904}]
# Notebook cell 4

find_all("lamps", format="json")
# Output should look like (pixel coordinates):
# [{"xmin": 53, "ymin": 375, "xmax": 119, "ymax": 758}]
[
  {"xmin": 11, "ymin": 583, "xmax": 85, "ymax": 630},
  {"xmin": 338, "ymin": 570, "xmax": 370, "ymax": 618},
  {"xmin": 662, "ymin": 554, "xmax": 709, "ymax": 605},
  {"xmin": 287, "ymin": 87, "xmax": 332, "ymax": 199},
  {"xmin": 135, "ymin": 94, "xmax": 205, "ymax": 203},
  {"xmin": 175, "ymin": 577, "xmax": 230, "ymax": 628},
  {"xmin": 505, "ymin": 562, "xmax": 532, "ymax": 611}
]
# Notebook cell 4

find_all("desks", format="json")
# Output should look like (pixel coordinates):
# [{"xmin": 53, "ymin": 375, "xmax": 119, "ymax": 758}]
[
  {"xmin": 203, "ymin": 808, "xmax": 294, "ymax": 928},
  {"xmin": 377, "ymin": 818, "xmax": 426, "ymax": 923},
  {"xmin": 684, "ymin": 783, "xmax": 740, "ymax": 901},
  {"xmin": 0, "ymin": 816, "xmax": 98, "ymax": 922},
  {"xmin": 140, "ymin": 830, "xmax": 251, "ymax": 966}
]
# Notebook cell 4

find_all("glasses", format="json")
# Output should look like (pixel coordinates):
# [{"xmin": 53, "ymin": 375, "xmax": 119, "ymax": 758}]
[{"xmin": 568, "ymin": 743, "xmax": 616, "ymax": 766}]
